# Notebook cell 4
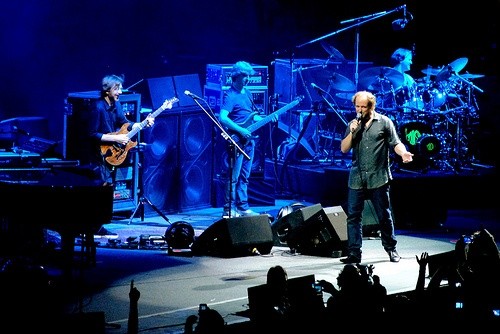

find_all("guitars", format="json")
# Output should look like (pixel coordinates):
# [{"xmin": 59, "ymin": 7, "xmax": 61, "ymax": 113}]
[
  {"xmin": 100, "ymin": 97, "xmax": 180, "ymax": 166},
  {"xmin": 225, "ymin": 95, "xmax": 305, "ymax": 159}
]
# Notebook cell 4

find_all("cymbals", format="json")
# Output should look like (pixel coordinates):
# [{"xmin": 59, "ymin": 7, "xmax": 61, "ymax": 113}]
[
  {"xmin": 359, "ymin": 67, "xmax": 404, "ymax": 92},
  {"xmin": 422, "ymin": 69, "xmax": 441, "ymax": 76},
  {"xmin": 451, "ymin": 74, "xmax": 484, "ymax": 82},
  {"xmin": 320, "ymin": 41, "xmax": 346, "ymax": 62},
  {"xmin": 436, "ymin": 57, "xmax": 468, "ymax": 82},
  {"xmin": 310, "ymin": 69, "xmax": 356, "ymax": 92}
]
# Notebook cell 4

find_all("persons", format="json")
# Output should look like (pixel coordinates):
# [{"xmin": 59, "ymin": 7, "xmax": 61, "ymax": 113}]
[
  {"xmin": 391, "ymin": 48, "xmax": 424, "ymax": 112},
  {"xmin": 219, "ymin": 61, "xmax": 279, "ymax": 217},
  {"xmin": 126, "ymin": 229, "xmax": 500, "ymax": 334},
  {"xmin": 340, "ymin": 90, "xmax": 414, "ymax": 263},
  {"xmin": 87, "ymin": 74, "xmax": 155, "ymax": 235}
]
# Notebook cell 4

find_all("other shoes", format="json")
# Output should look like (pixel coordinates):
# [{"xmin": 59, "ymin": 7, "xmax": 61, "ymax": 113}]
[
  {"xmin": 238, "ymin": 209, "xmax": 260, "ymax": 216},
  {"xmin": 222, "ymin": 211, "xmax": 241, "ymax": 218},
  {"xmin": 93, "ymin": 227, "xmax": 118, "ymax": 239},
  {"xmin": 340, "ymin": 255, "xmax": 362, "ymax": 263},
  {"xmin": 388, "ymin": 249, "xmax": 400, "ymax": 262}
]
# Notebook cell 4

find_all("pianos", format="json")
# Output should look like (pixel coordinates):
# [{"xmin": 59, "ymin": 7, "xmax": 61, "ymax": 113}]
[{"xmin": 0, "ymin": 165, "xmax": 114, "ymax": 271}]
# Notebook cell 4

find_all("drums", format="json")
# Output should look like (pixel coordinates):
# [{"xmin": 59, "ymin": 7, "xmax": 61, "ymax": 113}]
[{"xmin": 391, "ymin": 78, "xmax": 446, "ymax": 174}]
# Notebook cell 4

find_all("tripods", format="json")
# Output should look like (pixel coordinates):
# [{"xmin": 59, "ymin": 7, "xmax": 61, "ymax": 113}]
[
  {"xmin": 308, "ymin": 68, "xmax": 482, "ymax": 176},
  {"xmin": 128, "ymin": 132, "xmax": 171, "ymax": 228}
]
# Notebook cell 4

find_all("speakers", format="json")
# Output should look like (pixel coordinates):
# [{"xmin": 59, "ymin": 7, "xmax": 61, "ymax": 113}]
[
  {"xmin": 204, "ymin": 59, "xmax": 374, "ymax": 178},
  {"xmin": 178, "ymin": 109, "xmax": 214, "ymax": 214},
  {"xmin": 361, "ymin": 200, "xmax": 380, "ymax": 236},
  {"xmin": 192, "ymin": 214, "xmax": 274, "ymax": 258},
  {"xmin": 289, "ymin": 206, "xmax": 351, "ymax": 256},
  {"xmin": 143, "ymin": 112, "xmax": 178, "ymax": 218},
  {"xmin": 271, "ymin": 202, "xmax": 323, "ymax": 245}
]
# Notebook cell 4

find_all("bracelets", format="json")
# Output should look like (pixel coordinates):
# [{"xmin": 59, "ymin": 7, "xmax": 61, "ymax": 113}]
[{"xmin": 350, "ymin": 131, "xmax": 353, "ymax": 134}]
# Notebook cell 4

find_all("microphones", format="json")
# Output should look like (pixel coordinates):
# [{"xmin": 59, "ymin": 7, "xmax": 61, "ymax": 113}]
[
  {"xmin": 309, "ymin": 83, "xmax": 329, "ymax": 93},
  {"xmin": 351, "ymin": 113, "xmax": 362, "ymax": 133},
  {"xmin": 184, "ymin": 90, "xmax": 204, "ymax": 101}
]
